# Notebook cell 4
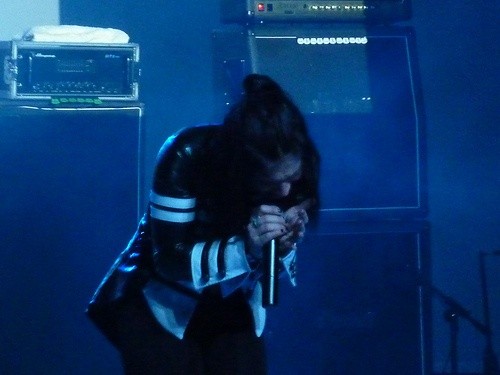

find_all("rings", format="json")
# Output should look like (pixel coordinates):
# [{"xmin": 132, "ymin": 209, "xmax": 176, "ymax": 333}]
[{"xmin": 251, "ymin": 215, "xmax": 263, "ymax": 227}]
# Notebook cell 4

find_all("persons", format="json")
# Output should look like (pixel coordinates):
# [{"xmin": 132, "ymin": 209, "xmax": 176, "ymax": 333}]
[{"xmin": 85, "ymin": 72, "xmax": 323, "ymax": 375}]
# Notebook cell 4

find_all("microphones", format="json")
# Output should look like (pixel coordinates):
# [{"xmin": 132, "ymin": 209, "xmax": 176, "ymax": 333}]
[{"xmin": 258, "ymin": 197, "xmax": 279, "ymax": 306}]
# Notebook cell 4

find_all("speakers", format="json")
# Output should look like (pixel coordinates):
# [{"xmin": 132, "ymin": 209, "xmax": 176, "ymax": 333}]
[
  {"xmin": 252, "ymin": 220, "xmax": 434, "ymax": 375},
  {"xmin": 1, "ymin": 102, "xmax": 143, "ymax": 375},
  {"xmin": 213, "ymin": 25, "xmax": 426, "ymax": 217}
]
